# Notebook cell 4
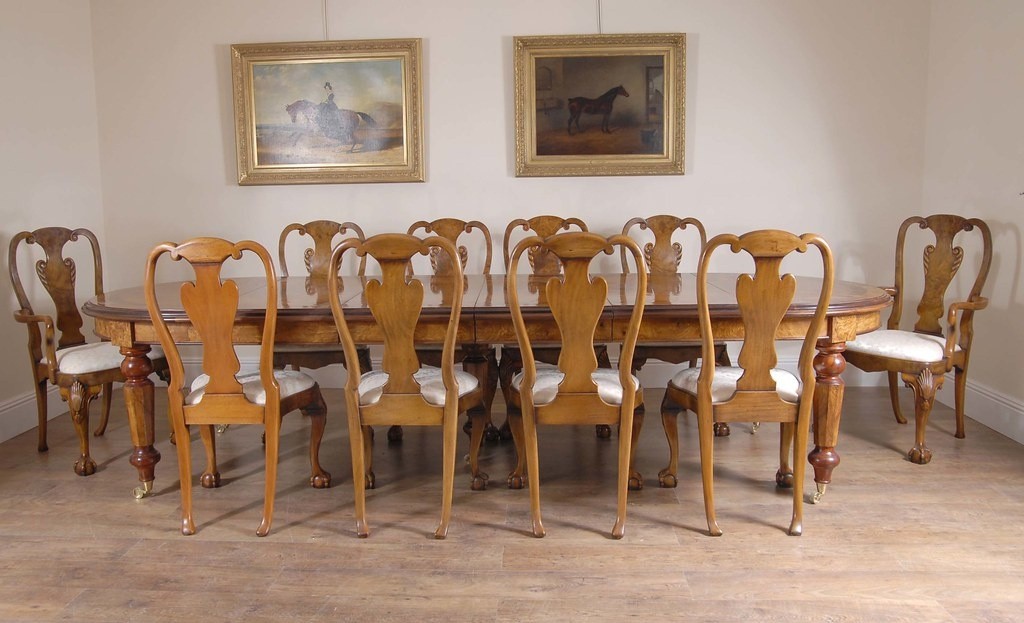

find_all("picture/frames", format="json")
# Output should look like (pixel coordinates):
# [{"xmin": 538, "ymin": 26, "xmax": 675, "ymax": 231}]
[
  {"xmin": 230, "ymin": 38, "xmax": 425, "ymax": 186},
  {"xmin": 514, "ymin": 35, "xmax": 687, "ymax": 177}
]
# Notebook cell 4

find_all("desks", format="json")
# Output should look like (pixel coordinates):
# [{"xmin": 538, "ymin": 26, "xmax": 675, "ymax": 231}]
[{"xmin": 80, "ymin": 272, "xmax": 896, "ymax": 504}]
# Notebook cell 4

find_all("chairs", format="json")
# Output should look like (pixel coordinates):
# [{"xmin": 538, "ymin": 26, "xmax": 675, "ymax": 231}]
[
  {"xmin": 327, "ymin": 234, "xmax": 489, "ymax": 540},
  {"xmin": 144, "ymin": 238, "xmax": 332, "ymax": 538},
  {"xmin": 388, "ymin": 216, "xmax": 498, "ymax": 444},
  {"xmin": 617, "ymin": 216, "xmax": 732, "ymax": 437},
  {"xmin": 656, "ymin": 229, "xmax": 834, "ymax": 536},
  {"xmin": 812, "ymin": 214, "xmax": 993, "ymax": 464},
  {"xmin": 495, "ymin": 216, "xmax": 613, "ymax": 442},
  {"xmin": 9, "ymin": 226, "xmax": 183, "ymax": 476},
  {"xmin": 259, "ymin": 221, "xmax": 378, "ymax": 440},
  {"xmin": 500, "ymin": 232, "xmax": 648, "ymax": 541}
]
